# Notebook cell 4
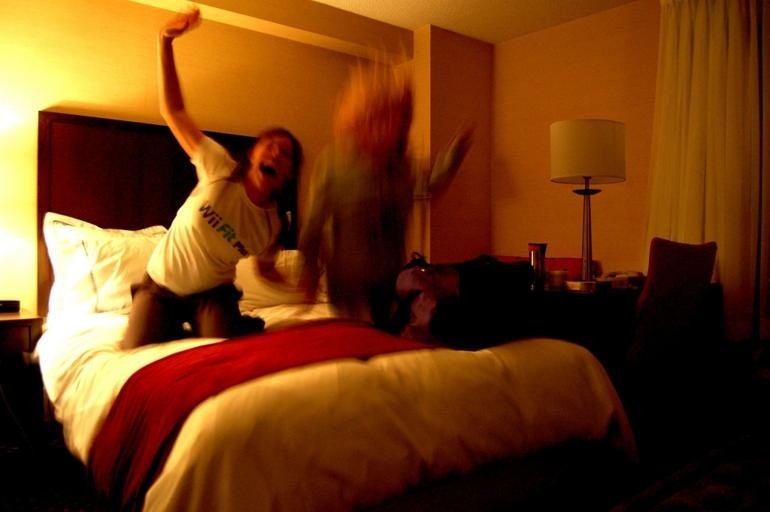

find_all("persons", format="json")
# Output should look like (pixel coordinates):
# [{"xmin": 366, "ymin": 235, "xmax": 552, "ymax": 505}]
[
  {"xmin": 286, "ymin": 47, "xmax": 482, "ymax": 323},
  {"xmin": 121, "ymin": 6, "xmax": 328, "ymax": 350}
]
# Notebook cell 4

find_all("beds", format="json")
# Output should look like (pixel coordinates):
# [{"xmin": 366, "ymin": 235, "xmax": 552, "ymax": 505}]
[{"xmin": 33, "ymin": 111, "xmax": 636, "ymax": 512}]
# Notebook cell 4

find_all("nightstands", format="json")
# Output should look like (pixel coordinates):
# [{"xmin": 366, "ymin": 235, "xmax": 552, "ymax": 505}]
[{"xmin": 0, "ymin": 317, "xmax": 44, "ymax": 457}]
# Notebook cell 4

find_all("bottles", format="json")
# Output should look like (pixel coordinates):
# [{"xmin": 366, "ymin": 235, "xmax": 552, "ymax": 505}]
[{"xmin": 527, "ymin": 243, "xmax": 547, "ymax": 292}]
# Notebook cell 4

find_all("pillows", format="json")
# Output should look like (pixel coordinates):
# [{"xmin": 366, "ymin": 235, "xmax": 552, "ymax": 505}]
[
  {"xmin": 235, "ymin": 251, "xmax": 331, "ymax": 314},
  {"xmin": 42, "ymin": 212, "xmax": 167, "ymax": 331},
  {"xmin": 625, "ymin": 236, "xmax": 718, "ymax": 365}
]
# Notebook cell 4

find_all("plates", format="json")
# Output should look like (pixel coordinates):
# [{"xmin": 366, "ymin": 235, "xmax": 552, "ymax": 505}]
[{"xmin": 566, "ymin": 281, "xmax": 596, "ymax": 293}]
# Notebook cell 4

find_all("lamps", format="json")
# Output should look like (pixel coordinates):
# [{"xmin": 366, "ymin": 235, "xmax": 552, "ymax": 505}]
[{"xmin": 549, "ymin": 117, "xmax": 626, "ymax": 281}]
